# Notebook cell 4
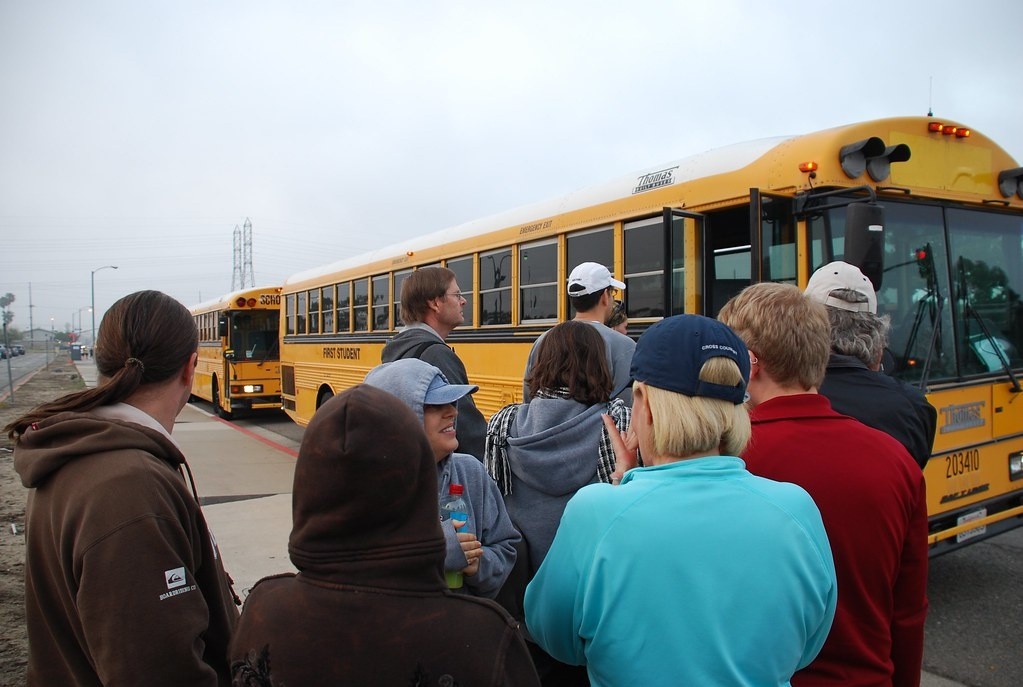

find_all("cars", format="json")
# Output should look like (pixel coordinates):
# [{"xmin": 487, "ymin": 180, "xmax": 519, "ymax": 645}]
[{"xmin": 0, "ymin": 343, "xmax": 26, "ymax": 360}]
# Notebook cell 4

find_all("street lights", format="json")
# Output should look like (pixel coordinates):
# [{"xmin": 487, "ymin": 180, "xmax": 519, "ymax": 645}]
[
  {"xmin": 90, "ymin": 265, "xmax": 119, "ymax": 352},
  {"xmin": 486, "ymin": 250, "xmax": 528, "ymax": 323},
  {"xmin": 78, "ymin": 305, "xmax": 92, "ymax": 337}
]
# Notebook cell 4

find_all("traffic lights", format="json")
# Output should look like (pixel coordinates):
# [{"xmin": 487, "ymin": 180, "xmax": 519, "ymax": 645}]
[{"xmin": 915, "ymin": 246, "xmax": 932, "ymax": 278}]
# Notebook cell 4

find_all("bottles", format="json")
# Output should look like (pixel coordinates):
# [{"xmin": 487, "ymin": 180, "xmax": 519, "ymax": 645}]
[{"xmin": 438, "ymin": 484, "xmax": 469, "ymax": 589}]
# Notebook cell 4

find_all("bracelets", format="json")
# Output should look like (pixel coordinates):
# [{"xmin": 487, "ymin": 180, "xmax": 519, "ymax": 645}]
[{"xmin": 609, "ymin": 474, "xmax": 621, "ymax": 481}]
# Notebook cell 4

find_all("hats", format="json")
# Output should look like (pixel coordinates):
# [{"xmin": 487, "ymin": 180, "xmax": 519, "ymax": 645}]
[
  {"xmin": 804, "ymin": 261, "xmax": 877, "ymax": 316},
  {"xmin": 566, "ymin": 262, "xmax": 626, "ymax": 297},
  {"xmin": 610, "ymin": 314, "xmax": 751, "ymax": 401},
  {"xmin": 424, "ymin": 374, "xmax": 480, "ymax": 405}
]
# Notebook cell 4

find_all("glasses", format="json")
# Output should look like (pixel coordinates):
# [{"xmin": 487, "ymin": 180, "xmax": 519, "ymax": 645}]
[
  {"xmin": 442, "ymin": 291, "xmax": 462, "ymax": 300},
  {"xmin": 608, "ymin": 300, "xmax": 625, "ymax": 326},
  {"xmin": 607, "ymin": 288, "xmax": 619, "ymax": 297}
]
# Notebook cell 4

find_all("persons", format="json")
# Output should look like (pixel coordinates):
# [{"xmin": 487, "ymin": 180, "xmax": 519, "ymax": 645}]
[
  {"xmin": 365, "ymin": 359, "xmax": 523, "ymax": 604},
  {"xmin": 524, "ymin": 261, "xmax": 639, "ymax": 410},
  {"xmin": 604, "ymin": 299, "xmax": 629, "ymax": 335},
  {"xmin": 81, "ymin": 346, "xmax": 93, "ymax": 358},
  {"xmin": 381, "ymin": 267, "xmax": 487, "ymax": 462},
  {"xmin": 2, "ymin": 290, "xmax": 242, "ymax": 687},
  {"xmin": 229, "ymin": 385, "xmax": 542, "ymax": 687},
  {"xmin": 718, "ymin": 283, "xmax": 930, "ymax": 687},
  {"xmin": 804, "ymin": 260, "xmax": 937, "ymax": 471},
  {"xmin": 482, "ymin": 321, "xmax": 632, "ymax": 580},
  {"xmin": 522, "ymin": 314, "xmax": 838, "ymax": 687}
]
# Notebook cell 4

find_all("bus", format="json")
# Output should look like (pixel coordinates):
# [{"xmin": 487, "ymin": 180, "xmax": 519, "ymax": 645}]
[
  {"xmin": 187, "ymin": 284, "xmax": 283, "ymax": 422},
  {"xmin": 276, "ymin": 114, "xmax": 1023, "ymax": 559}
]
